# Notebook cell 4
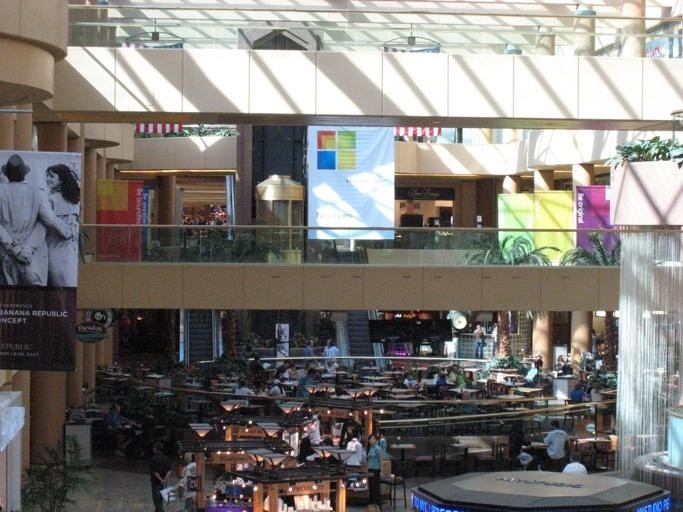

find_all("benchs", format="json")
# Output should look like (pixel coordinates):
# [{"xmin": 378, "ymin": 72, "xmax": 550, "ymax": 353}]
[{"xmin": 84, "ymin": 358, "xmax": 618, "ymax": 476}]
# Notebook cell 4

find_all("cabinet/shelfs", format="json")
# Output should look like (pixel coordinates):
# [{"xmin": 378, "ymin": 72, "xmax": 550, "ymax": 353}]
[{"xmin": 64, "ymin": 415, "xmax": 92, "ymax": 467}]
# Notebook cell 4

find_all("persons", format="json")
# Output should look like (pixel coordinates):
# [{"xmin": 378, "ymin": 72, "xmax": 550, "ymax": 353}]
[{"xmin": 0, "ymin": 154, "xmax": 81, "ymax": 288}]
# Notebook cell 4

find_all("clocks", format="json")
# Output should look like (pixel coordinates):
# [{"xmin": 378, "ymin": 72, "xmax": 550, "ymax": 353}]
[{"xmin": 452, "ymin": 312, "xmax": 468, "ymax": 330}]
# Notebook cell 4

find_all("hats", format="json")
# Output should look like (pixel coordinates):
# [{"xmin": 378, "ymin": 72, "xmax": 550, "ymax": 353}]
[{"xmin": 1, "ymin": 154, "xmax": 31, "ymax": 179}]
